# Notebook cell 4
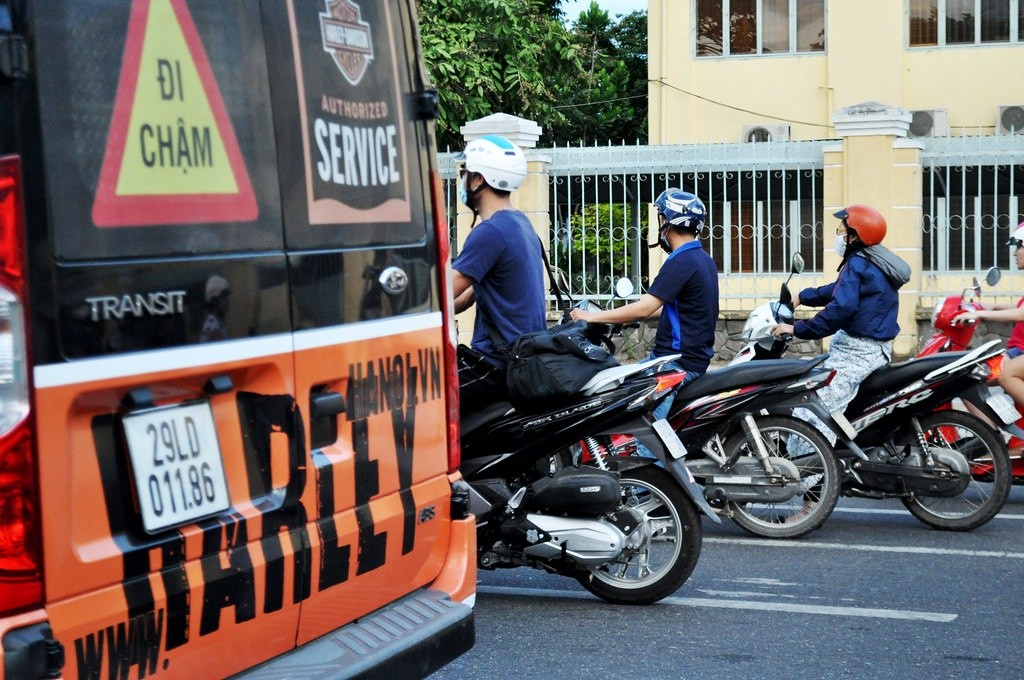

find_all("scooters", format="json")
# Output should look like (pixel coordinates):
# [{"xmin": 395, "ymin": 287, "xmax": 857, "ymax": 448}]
[{"xmin": 908, "ymin": 267, "xmax": 1024, "ymax": 485}]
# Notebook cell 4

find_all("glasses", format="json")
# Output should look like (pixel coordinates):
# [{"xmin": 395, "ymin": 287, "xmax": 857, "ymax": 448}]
[
  {"xmin": 460, "ymin": 164, "xmax": 468, "ymax": 177},
  {"xmin": 836, "ymin": 227, "xmax": 848, "ymax": 235}
]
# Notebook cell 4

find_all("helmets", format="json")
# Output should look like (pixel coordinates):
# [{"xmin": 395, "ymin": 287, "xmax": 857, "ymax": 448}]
[
  {"xmin": 463, "ymin": 136, "xmax": 527, "ymax": 192},
  {"xmin": 834, "ymin": 205, "xmax": 886, "ymax": 247},
  {"xmin": 1005, "ymin": 221, "xmax": 1024, "ymax": 247},
  {"xmin": 653, "ymin": 188, "xmax": 706, "ymax": 231}
]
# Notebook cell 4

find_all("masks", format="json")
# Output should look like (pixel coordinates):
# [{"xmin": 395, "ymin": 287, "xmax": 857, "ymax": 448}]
[
  {"xmin": 460, "ymin": 179, "xmax": 478, "ymax": 206},
  {"xmin": 834, "ymin": 234, "xmax": 856, "ymax": 256},
  {"xmin": 661, "ymin": 225, "xmax": 669, "ymax": 248}
]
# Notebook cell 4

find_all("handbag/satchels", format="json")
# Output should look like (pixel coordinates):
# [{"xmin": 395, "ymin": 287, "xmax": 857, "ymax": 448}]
[{"xmin": 507, "ymin": 321, "xmax": 621, "ymax": 403}]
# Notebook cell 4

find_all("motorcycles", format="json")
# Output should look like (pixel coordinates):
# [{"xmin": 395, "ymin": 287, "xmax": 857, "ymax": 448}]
[
  {"xmin": 462, "ymin": 353, "xmax": 723, "ymax": 606},
  {"xmin": 720, "ymin": 251, "xmax": 1024, "ymax": 532},
  {"xmin": 548, "ymin": 266, "xmax": 870, "ymax": 540}
]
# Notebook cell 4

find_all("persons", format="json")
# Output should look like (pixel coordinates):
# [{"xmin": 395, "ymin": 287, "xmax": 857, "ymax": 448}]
[
  {"xmin": 569, "ymin": 188, "xmax": 720, "ymax": 519},
  {"xmin": 952, "ymin": 221, "xmax": 1024, "ymax": 408},
  {"xmin": 451, "ymin": 136, "xmax": 547, "ymax": 408},
  {"xmin": 771, "ymin": 205, "xmax": 912, "ymax": 529}
]
